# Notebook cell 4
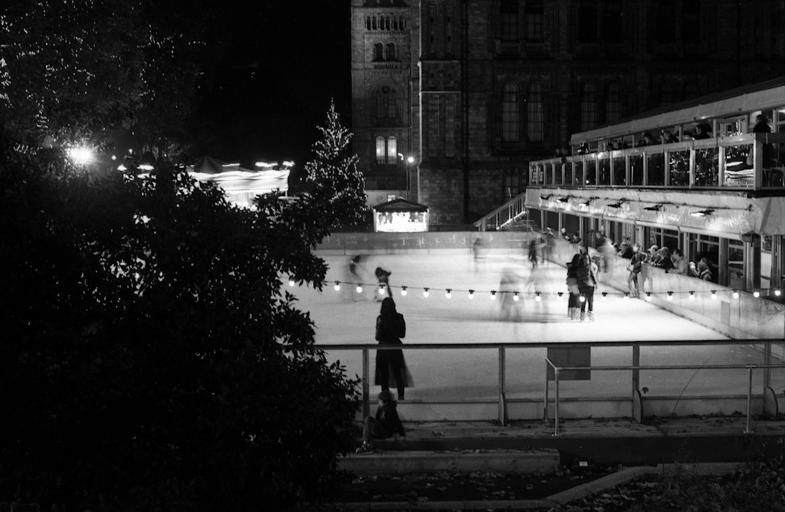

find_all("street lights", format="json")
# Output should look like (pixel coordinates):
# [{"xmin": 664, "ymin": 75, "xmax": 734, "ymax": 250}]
[{"xmin": 405, "ymin": 156, "xmax": 415, "ymax": 201}]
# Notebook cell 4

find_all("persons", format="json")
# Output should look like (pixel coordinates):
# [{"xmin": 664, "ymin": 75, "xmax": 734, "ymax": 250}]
[
  {"xmin": 339, "ymin": 254, "xmax": 362, "ymax": 303},
  {"xmin": 373, "ymin": 265, "xmax": 393, "ymax": 302},
  {"xmin": 355, "ymin": 389, "xmax": 398, "ymax": 454},
  {"xmin": 372, "ymin": 297, "xmax": 417, "ymax": 399},
  {"xmin": 469, "ymin": 225, "xmax": 717, "ymax": 326},
  {"xmin": 545, "ymin": 113, "xmax": 785, "ymax": 187}
]
